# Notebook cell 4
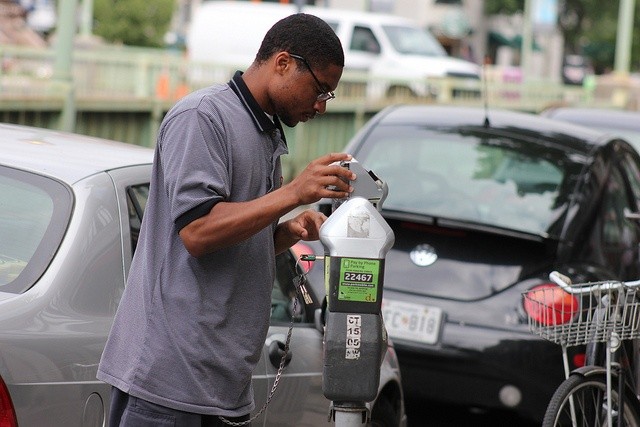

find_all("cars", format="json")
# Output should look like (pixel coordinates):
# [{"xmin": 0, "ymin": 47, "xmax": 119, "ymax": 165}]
[
  {"xmin": 543, "ymin": 108, "xmax": 639, "ymax": 139},
  {"xmin": 311, "ymin": 102, "xmax": 640, "ymax": 426},
  {"xmin": 0, "ymin": 122, "xmax": 411, "ymax": 427}
]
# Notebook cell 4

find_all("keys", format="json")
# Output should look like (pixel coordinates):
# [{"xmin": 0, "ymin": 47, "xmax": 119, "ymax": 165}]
[{"xmin": 292, "ymin": 254, "xmax": 325, "ymax": 305}]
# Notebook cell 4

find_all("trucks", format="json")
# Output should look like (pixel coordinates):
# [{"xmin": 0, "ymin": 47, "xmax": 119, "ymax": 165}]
[{"xmin": 186, "ymin": 1, "xmax": 484, "ymax": 102}]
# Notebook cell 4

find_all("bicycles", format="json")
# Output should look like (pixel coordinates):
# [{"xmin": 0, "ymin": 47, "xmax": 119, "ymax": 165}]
[{"xmin": 520, "ymin": 272, "xmax": 640, "ymax": 427}]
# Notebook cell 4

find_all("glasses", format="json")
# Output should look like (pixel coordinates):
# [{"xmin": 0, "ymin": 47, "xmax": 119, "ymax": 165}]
[{"xmin": 289, "ymin": 53, "xmax": 336, "ymax": 102}]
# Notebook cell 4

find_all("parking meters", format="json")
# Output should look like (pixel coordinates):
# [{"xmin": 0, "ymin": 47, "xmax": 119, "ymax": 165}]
[{"xmin": 317, "ymin": 153, "xmax": 395, "ymax": 427}]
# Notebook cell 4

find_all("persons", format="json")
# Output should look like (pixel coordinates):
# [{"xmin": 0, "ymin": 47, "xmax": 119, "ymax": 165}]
[{"xmin": 96, "ymin": 14, "xmax": 358, "ymax": 426}]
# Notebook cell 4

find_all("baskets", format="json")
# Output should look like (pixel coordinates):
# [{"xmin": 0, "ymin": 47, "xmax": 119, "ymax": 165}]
[{"xmin": 519, "ymin": 279, "xmax": 640, "ymax": 347}]
